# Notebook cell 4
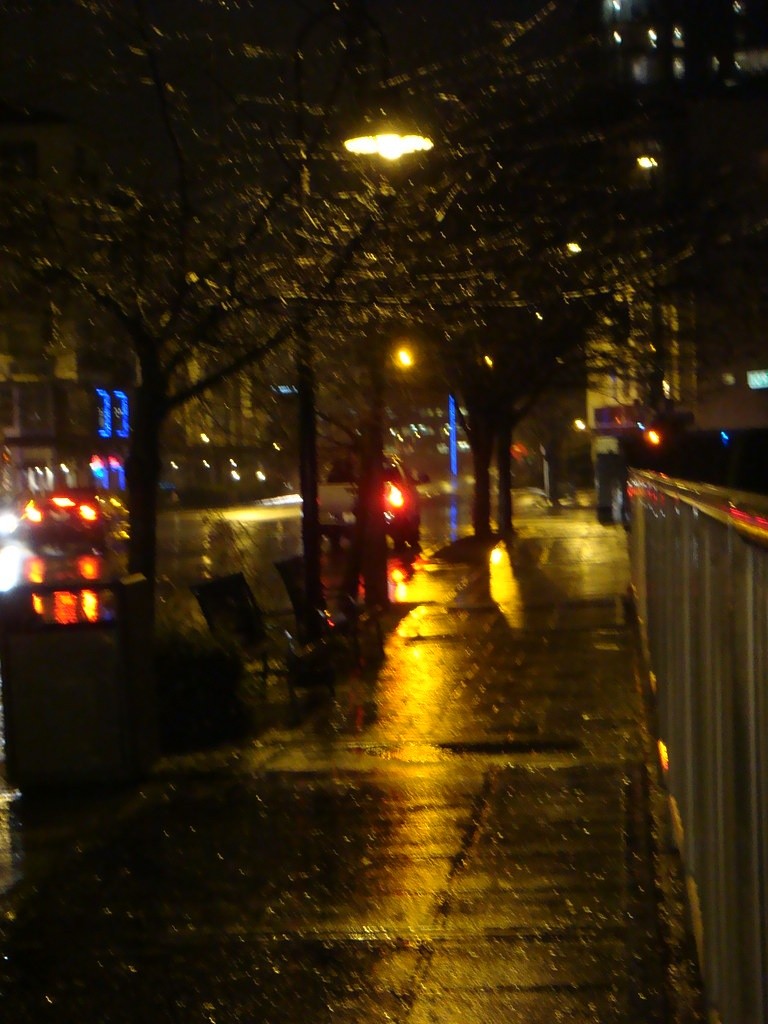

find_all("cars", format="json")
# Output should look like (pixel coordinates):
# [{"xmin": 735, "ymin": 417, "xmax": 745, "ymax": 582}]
[
  {"xmin": 18, "ymin": 486, "xmax": 112, "ymax": 544},
  {"xmin": 300, "ymin": 450, "xmax": 432, "ymax": 549}
]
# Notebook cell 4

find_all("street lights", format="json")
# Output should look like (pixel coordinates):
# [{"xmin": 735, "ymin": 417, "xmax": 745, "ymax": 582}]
[{"xmin": 290, "ymin": 3, "xmax": 436, "ymax": 675}]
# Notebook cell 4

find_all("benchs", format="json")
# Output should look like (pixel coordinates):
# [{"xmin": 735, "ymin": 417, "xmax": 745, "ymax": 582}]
[
  {"xmin": 189, "ymin": 573, "xmax": 340, "ymax": 715},
  {"xmin": 272, "ymin": 556, "xmax": 389, "ymax": 699}
]
